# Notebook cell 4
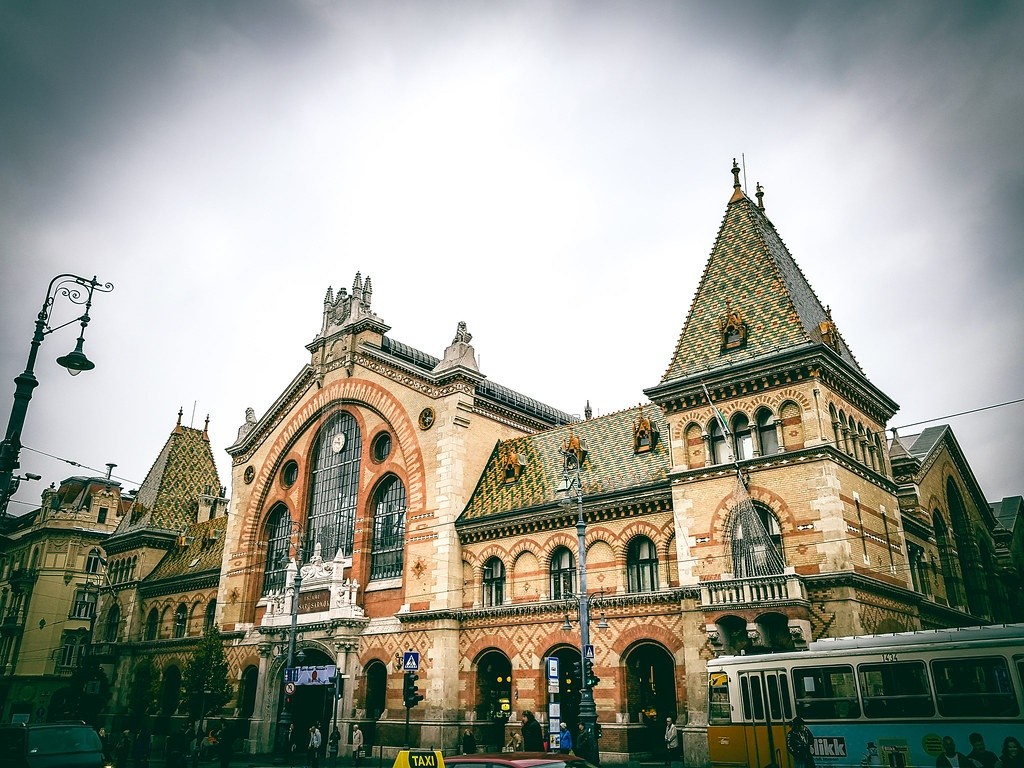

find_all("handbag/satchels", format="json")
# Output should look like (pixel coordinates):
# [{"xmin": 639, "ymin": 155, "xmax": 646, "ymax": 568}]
[
  {"xmin": 328, "ymin": 745, "xmax": 337, "ymax": 752},
  {"xmin": 356, "ymin": 746, "xmax": 365, "ymax": 758}
]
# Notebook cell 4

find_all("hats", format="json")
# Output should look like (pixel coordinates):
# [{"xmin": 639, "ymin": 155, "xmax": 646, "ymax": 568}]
[{"xmin": 560, "ymin": 722, "xmax": 566, "ymax": 729}]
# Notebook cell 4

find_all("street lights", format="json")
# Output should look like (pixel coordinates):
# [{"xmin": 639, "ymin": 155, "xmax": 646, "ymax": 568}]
[
  {"xmin": 0, "ymin": 273, "xmax": 115, "ymax": 527},
  {"xmin": 556, "ymin": 445, "xmax": 611, "ymax": 765},
  {"xmin": 272, "ymin": 534, "xmax": 306, "ymax": 765},
  {"xmin": 76, "ymin": 579, "xmax": 102, "ymax": 713}
]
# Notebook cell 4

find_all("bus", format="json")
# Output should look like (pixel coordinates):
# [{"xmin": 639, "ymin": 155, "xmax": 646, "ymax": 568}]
[{"xmin": 704, "ymin": 620, "xmax": 1024, "ymax": 768}]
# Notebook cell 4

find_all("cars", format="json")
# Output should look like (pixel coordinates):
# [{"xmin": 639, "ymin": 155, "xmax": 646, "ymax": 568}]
[
  {"xmin": 0, "ymin": 718, "xmax": 107, "ymax": 768},
  {"xmin": 444, "ymin": 749, "xmax": 599, "ymax": 768}
]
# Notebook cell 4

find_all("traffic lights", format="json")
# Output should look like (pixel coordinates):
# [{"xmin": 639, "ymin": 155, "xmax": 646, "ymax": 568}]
[
  {"xmin": 573, "ymin": 661, "xmax": 582, "ymax": 677},
  {"xmin": 586, "ymin": 659, "xmax": 594, "ymax": 678},
  {"xmin": 403, "ymin": 672, "xmax": 419, "ymax": 708},
  {"xmin": 328, "ymin": 675, "xmax": 338, "ymax": 694}
]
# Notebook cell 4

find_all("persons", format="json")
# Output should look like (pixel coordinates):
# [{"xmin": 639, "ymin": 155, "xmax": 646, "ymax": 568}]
[
  {"xmin": 463, "ymin": 729, "xmax": 478, "ymax": 755},
  {"xmin": 560, "ymin": 722, "xmax": 572, "ymax": 755},
  {"xmin": 577, "ymin": 721, "xmax": 590, "ymax": 756},
  {"xmin": 506, "ymin": 731, "xmax": 522, "ymax": 752},
  {"xmin": 521, "ymin": 710, "xmax": 546, "ymax": 752},
  {"xmin": 286, "ymin": 721, "xmax": 364, "ymax": 768},
  {"xmin": 98, "ymin": 722, "xmax": 230, "ymax": 768},
  {"xmin": 664, "ymin": 717, "xmax": 679, "ymax": 761}
]
